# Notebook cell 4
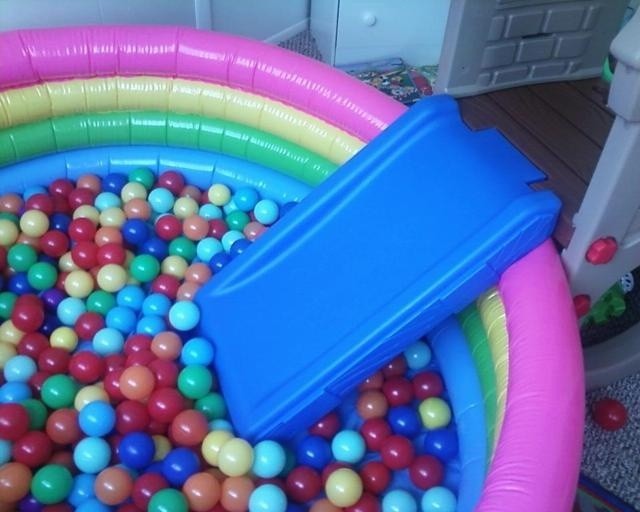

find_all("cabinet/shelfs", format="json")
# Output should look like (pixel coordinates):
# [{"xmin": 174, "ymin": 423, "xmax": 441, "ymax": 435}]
[{"xmin": 310, "ymin": 0, "xmax": 451, "ymax": 74}]
